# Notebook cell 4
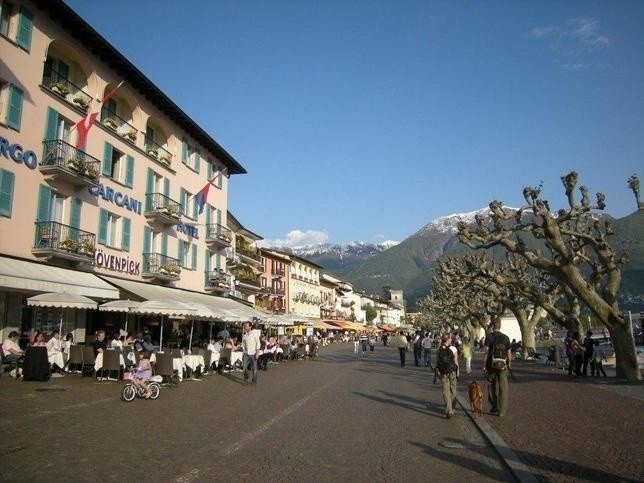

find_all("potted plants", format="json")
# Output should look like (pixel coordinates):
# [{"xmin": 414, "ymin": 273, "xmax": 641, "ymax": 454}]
[
  {"xmin": 160, "ymin": 158, "xmax": 170, "ymax": 166},
  {"xmin": 147, "ymin": 147, "xmax": 159, "ymax": 159},
  {"xmin": 154, "ymin": 205, "xmax": 181, "ymax": 219},
  {"xmin": 72, "ymin": 98, "xmax": 89, "ymax": 111},
  {"xmin": 104, "ymin": 117, "xmax": 118, "ymax": 129},
  {"xmin": 158, "ymin": 264, "xmax": 181, "ymax": 276},
  {"xmin": 68, "ymin": 159, "xmax": 98, "ymax": 179},
  {"xmin": 50, "ymin": 83, "xmax": 70, "ymax": 98},
  {"xmin": 58, "ymin": 239, "xmax": 95, "ymax": 258},
  {"xmin": 219, "ymin": 233, "xmax": 231, "ymax": 243},
  {"xmin": 123, "ymin": 132, "xmax": 136, "ymax": 144}
]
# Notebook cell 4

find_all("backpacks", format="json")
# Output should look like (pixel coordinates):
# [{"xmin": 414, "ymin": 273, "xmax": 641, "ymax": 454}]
[
  {"xmin": 491, "ymin": 333, "xmax": 508, "ymax": 370},
  {"xmin": 436, "ymin": 344, "xmax": 456, "ymax": 374}
]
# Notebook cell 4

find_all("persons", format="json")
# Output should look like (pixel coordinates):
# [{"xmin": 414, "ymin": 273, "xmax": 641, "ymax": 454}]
[
  {"xmin": 134, "ymin": 351, "xmax": 153, "ymax": 399},
  {"xmin": 396, "ymin": 329, "xmax": 442, "ymax": 367},
  {"xmin": 450, "ymin": 335, "xmax": 484, "ymax": 373},
  {"xmin": 93, "ymin": 325, "xmax": 242, "ymax": 381},
  {"xmin": 322, "ymin": 329, "xmax": 355, "ymax": 346},
  {"xmin": 1, "ymin": 329, "xmax": 82, "ymax": 378},
  {"xmin": 354, "ymin": 332, "xmax": 391, "ymax": 358},
  {"xmin": 436, "ymin": 332, "xmax": 459, "ymax": 418},
  {"xmin": 512, "ymin": 339, "xmax": 525, "ymax": 360},
  {"xmin": 539, "ymin": 328, "xmax": 555, "ymax": 340},
  {"xmin": 564, "ymin": 329, "xmax": 606, "ymax": 379},
  {"xmin": 257, "ymin": 329, "xmax": 320, "ymax": 371},
  {"xmin": 242, "ymin": 322, "xmax": 261, "ymax": 385},
  {"xmin": 482, "ymin": 319, "xmax": 512, "ymax": 417}
]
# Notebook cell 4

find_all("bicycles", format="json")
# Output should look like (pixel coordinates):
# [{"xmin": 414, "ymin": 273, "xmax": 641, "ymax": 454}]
[{"xmin": 121, "ymin": 366, "xmax": 160, "ymax": 400}]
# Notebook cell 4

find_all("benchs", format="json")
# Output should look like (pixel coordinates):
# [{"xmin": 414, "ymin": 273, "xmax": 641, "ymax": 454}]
[
  {"xmin": 546, "ymin": 355, "xmax": 568, "ymax": 367},
  {"xmin": 526, "ymin": 347, "xmax": 543, "ymax": 361}
]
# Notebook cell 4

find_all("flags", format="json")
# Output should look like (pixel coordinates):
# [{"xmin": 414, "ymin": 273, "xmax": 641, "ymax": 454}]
[
  {"xmin": 75, "ymin": 87, "xmax": 118, "ymax": 148},
  {"xmin": 195, "ymin": 175, "xmax": 218, "ymax": 214}
]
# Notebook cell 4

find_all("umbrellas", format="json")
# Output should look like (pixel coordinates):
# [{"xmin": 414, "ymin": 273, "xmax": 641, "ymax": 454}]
[{"xmin": 26, "ymin": 290, "xmax": 314, "ymax": 353}]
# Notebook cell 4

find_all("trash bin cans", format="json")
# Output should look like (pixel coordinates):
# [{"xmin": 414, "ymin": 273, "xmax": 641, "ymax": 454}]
[{"xmin": 549, "ymin": 345, "xmax": 559, "ymax": 367}]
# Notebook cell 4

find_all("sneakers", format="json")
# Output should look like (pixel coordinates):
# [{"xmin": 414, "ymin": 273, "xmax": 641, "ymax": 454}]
[
  {"xmin": 145, "ymin": 391, "xmax": 152, "ymax": 398},
  {"xmin": 9, "ymin": 369, "xmax": 24, "ymax": 379},
  {"xmin": 244, "ymin": 377, "xmax": 249, "ymax": 386},
  {"xmin": 400, "ymin": 362, "xmax": 433, "ymax": 368},
  {"xmin": 447, "ymin": 409, "xmax": 456, "ymax": 419},
  {"xmin": 252, "ymin": 377, "xmax": 256, "ymax": 385},
  {"xmin": 488, "ymin": 408, "xmax": 505, "ymax": 417},
  {"xmin": 567, "ymin": 369, "xmax": 608, "ymax": 378}
]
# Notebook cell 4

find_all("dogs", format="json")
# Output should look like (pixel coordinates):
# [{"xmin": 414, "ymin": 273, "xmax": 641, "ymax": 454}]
[{"xmin": 468, "ymin": 380, "xmax": 484, "ymax": 420}]
